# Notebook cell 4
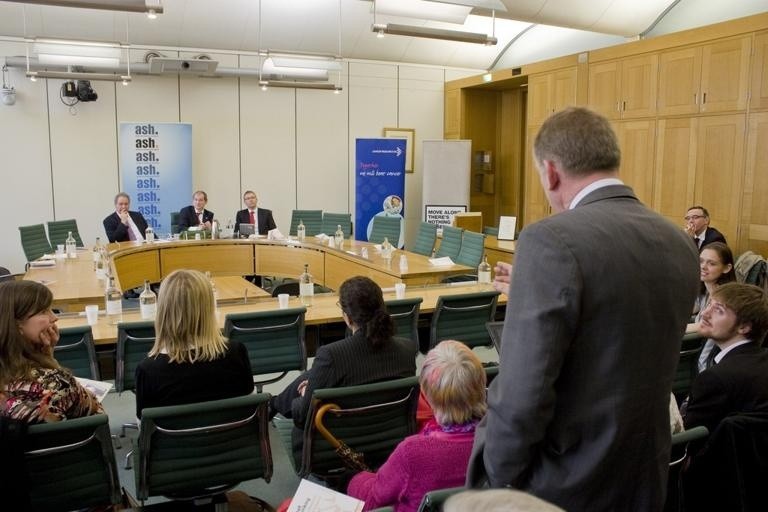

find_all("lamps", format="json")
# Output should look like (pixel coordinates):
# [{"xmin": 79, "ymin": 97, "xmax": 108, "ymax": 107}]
[
  {"xmin": 21, "ymin": 5, "xmax": 135, "ymax": 86},
  {"xmin": 0, "ymin": 0, "xmax": 166, "ymax": 21},
  {"xmin": 367, "ymin": 1, "xmax": 507, "ymax": 46},
  {"xmin": 255, "ymin": 0, "xmax": 346, "ymax": 94}
]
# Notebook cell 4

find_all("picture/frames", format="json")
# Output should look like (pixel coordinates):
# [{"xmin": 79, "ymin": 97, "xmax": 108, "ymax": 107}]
[{"xmin": 382, "ymin": 127, "xmax": 416, "ymax": 173}]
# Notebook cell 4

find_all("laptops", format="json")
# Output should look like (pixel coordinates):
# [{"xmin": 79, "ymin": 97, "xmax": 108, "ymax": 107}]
[{"xmin": 485, "ymin": 321, "xmax": 504, "ymax": 356}]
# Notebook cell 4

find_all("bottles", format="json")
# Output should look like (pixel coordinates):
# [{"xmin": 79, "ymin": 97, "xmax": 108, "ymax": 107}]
[
  {"xmin": 299, "ymin": 264, "xmax": 314, "ymax": 307},
  {"xmin": 335, "ymin": 225, "xmax": 344, "ymax": 246},
  {"xmin": 105, "ymin": 277, "xmax": 122, "ymax": 326},
  {"xmin": 139, "ymin": 280, "xmax": 157, "ymax": 320},
  {"xmin": 476, "ymin": 254, "xmax": 492, "ymax": 286},
  {"xmin": 297, "ymin": 218, "xmax": 306, "ymax": 241},
  {"xmin": 92, "ymin": 237, "xmax": 101, "ymax": 271},
  {"xmin": 212, "ymin": 220, "xmax": 218, "ymax": 239},
  {"xmin": 204, "ymin": 271, "xmax": 221, "ymax": 321},
  {"xmin": 381, "ymin": 237, "xmax": 392, "ymax": 258},
  {"xmin": 65, "ymin": 231, "xmax": 76, "ymax": 258},
  {"xmin": 145, "ymin": 224, "xmax": 154, "ymax": 242}
]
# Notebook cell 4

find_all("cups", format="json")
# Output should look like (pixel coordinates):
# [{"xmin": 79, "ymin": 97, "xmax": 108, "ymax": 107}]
[
  {"xmin": 56, "ymin": 250, "xmax": 64, "ymax": 268},
  {"xmin": 96, "ymin": 255, "xmax": 106, "ymax": 279},
  {"xmin": 328, "ymin": 236, "xmax": 335, "ymax": 249},
  {"xmin": 278, "ymin": 292, "xmax": 290, "ymax": 309},
  {"xmin": 85, "ymin": 305, "xmax": 99, "ymax": 325},
  {"xmin": 268, "ymin": 231, "xmax": 273, "ymax": 240},
  {"xmin": 56, "ymin": 245, "xmax": 64, "ymax": 250},
  {"xmin": 195, "ymin": 234, "xmax": 201, "ymax": 240},
  {"xmin": 395, "ymin": 283, "xmax": 407, "ymax": 299},
  {"xmin": 399, "ymin": 255, "xmax": 408, "ymax": 273},
  {"xmin": 361, "ymin": 247, "xmax": 369, "ymax": 260}
]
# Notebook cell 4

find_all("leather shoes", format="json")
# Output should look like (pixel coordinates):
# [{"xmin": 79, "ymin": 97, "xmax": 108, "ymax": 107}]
[{"xmin": 267, "ymin": 396, "xmax": 278, "ymax": 421}]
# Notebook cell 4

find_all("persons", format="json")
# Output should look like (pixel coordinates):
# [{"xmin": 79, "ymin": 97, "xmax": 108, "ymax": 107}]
[
  {"xmin": 103, "ymin": 193, "xmax": 160, "ymax": 300},
  {"xmin": 462, "ymin": 107, "xmax": 704, "ymax": 511},
  {"xmin": 135, "ymin": 270, "xmax": 257, "ymax": 421},
  {"xmin": 344, "ymin": 336, "xmax": 487, "ymax": 511},
  {"xmin": 441, "ymin": 487, "xmax": 566, "ymax": 512},
  {"xmin": 661, "ymin": 390, "xmax": 695, "ymax": 477},
  {"xmin": 682, "ymin": 284, "xmax": 765, "ymax": 441},
  {"xmin": 1, "ymin": 278, "xmax": 107, "ymax": 424},
  {"xmin": 266, "ymin": 274, "xmax": 419, "ymax": 477},
  {"xmin": 683, "ymin": 242, "xmax": 736, "ymax": 336},
  {"xmin": 681, "ymin": 205, "xmax": 728, "ymax": 253},
  {"xmin": 178, "ymin": 190, "xmax": 214, "ymax": 237},
  {"xmin": 232, "ymin": 192, "xmax": 277, "ymax": 287}
]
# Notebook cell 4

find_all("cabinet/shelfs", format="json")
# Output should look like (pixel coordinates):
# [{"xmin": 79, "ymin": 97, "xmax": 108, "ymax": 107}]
[
  {"xmin": 657, "ymin": 17, "xmax": 751, "ymax": 118},
  {"xmin": 606, "ymin": 117, "xmax": 657, "ymax": 215},
  {"xmin": 751, "ymin": 11, "xmax": 768, "ymax": 113},
  {"xmin": 588, "ymin": 37, "xmax": 657, "ymax": 117},
  {"xmin": 657, "ymin": 113, "xmax": 748, "ymax": 258},
  {"xmin": 748, "ymin": 113, "xmax": 768, "ymax": 259},
  {"xmin": 524, "ymin": 125, "xmax": 560, "ymax": 229},
  {"xmin": 526, "ymin": 52, "xmax": 579, "ymax": 124}
]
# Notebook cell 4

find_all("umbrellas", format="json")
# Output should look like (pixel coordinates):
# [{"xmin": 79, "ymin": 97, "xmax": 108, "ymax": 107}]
[{"xmin": 313, "ymin": 401, "xmax": 372, "ymax": 479}]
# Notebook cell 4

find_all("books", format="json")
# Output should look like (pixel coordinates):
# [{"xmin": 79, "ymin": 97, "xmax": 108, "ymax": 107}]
[{"xmin": 28, "ymin": 259, "xmax": 56, "ymax": 269}]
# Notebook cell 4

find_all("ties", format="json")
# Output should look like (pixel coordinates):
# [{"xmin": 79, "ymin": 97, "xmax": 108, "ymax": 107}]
[
  {"xmin": 694, "ymin": 238, "xmax": 700, "ymax": 246},
  {"xmin": 127, "ymin": 222, "xmax": 136, "ymax": 240},
  {"xmin": 249, "ymin": 212, "xmax": 255, "ymax": 224}
]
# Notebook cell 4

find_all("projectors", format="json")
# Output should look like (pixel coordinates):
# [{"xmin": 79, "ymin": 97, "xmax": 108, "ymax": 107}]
[{"xmin": 76, "ymin": 82, "xmax": 98, "ymax": 101}]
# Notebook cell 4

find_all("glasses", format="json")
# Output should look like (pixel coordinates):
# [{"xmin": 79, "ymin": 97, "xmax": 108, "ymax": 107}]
[
  {"xmin": 683, "ymin": 215, "xmax": 705, "ymax": 220},
  {"xmin": 336, "ymin": 301, "xmax": 344, "ymax": 311},
  {"xmin": 245, "ymin": 197, "xmax": 256, "ymax": 200}
]
